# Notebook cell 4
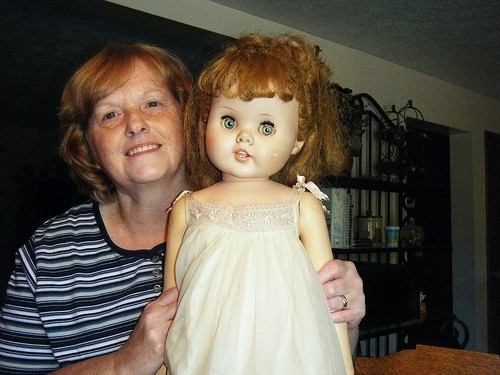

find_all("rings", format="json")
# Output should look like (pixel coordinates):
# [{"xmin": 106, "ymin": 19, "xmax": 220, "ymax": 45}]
[{"xmin": 340, "ymin": 295, "xmax": 349, "ymax": 308}]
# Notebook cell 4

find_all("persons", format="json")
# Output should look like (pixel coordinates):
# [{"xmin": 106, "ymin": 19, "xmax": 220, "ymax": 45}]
[
  {"xmin": 158, "ymin": 31, "xmax": 356, "ymax": 375},
  {"xmin": 0, "ymin": 38, "xmax": 366, "ymax": 375}
]
